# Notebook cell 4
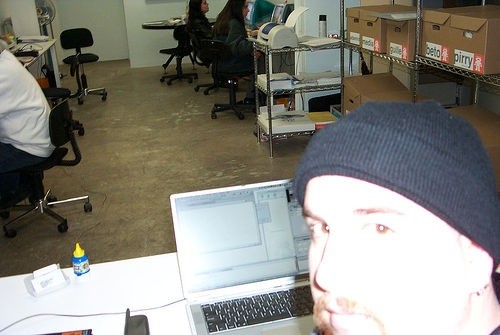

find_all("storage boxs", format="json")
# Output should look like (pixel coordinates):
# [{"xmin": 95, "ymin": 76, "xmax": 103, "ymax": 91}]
[{"xmin": 344, "ymin": 4, "xmax": 500, "ymax": 194}]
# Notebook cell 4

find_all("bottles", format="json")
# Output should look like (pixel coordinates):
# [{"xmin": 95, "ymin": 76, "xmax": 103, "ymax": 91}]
[
  {"xmin": 319, "ymin": 14, "xmax": 327, "ymax": 38},
  {"xmin": 71, "ymin": 242, "xmax": 90, "ymax": 277}
]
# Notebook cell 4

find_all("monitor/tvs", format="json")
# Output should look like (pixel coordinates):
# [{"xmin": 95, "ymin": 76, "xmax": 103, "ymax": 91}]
[
  {"xmin": 246, "ymin": 2, "xmax": 254, "ymax": 21},
  {"xmin": 270, "ymin": 4, "xmax": 285, "ymax": 23}
]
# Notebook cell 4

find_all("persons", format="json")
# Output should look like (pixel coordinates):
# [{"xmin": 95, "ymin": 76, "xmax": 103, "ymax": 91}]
[
  {"xmin": 188, "ymin": 0, "xmax": 213, "ymax": 62},
  {"xmin": 0, "ymin": 49, "xmax": 56, "ymax": 210},
  {"xmin": 209, "ymin": 0, "xmax": 261, "ymax": 104},
  {"xmin": 294, "ymin": 102, "xmax": 500, "ymax": 335}
]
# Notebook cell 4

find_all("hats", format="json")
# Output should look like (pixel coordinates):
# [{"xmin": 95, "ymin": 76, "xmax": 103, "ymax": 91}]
[{"xmin": 292, "ymin": 99, "xmax": 500, "ymax": 265}]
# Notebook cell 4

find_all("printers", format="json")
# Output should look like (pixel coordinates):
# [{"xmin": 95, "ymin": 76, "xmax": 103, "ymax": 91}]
[{"xmin": 257, "ymin": 7, "xmax": 308, "ymax": 50}]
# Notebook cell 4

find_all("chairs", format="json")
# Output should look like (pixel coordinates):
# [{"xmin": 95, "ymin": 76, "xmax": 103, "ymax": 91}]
[
  {"xmin": 60, "ymin": 28, "xmax": 107, "ymax": 105},
  {"xmin": 188, "ymin": 32, "xmax": 256, "ymax": 119},
  {"xmin": 160, "ymin": 25, "xmax": 198, "ymax": 86},
  {"xmin": 0, "ymin": 97, "xmax": 93, "ymax": 238}
]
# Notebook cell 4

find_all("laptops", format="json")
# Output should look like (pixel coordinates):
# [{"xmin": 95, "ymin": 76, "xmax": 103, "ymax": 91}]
[{"xmin": 170, "ymin": 179, "xmax": 319, "ymax": 335}]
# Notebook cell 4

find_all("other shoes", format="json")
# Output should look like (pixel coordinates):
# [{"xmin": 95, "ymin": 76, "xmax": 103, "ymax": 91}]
[
  {"xmin": 244, "ymin": 98, "xmax": 255, "ymax": 105},
  {"xmin": 252, "ymin": 107, "xmax": 256, "ymax": 112}
]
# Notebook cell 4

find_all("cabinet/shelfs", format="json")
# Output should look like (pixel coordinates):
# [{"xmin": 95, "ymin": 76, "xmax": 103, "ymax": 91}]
[
  {"xmin": 253, "ymin": 41, "xmax": 352, "ymax": 159},
  {"xmin": 340, "ymin": 0, "xmax": 500, "ymax": 117}
]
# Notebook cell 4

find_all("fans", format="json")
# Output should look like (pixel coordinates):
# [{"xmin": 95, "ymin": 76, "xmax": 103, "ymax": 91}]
[{"xmin": 35, "ymin": 0, "xmax": 56, "ymax": 71}]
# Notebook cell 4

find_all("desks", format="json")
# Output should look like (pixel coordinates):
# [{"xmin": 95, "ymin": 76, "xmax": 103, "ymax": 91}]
[
  {"xmin": 11, "ymin": 34, "xmax": 61, "ymax": 88},
  {"xmin": 142, "ymin": 18, "xmax": 216, "ymax": 29},
  {"xmin": 0, "ymin": 252, "xmax": 317, "ymax": 334}
]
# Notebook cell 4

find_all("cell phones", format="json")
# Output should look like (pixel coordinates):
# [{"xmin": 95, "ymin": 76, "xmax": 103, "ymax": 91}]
[{"xmin": 124, "ymin": 308, "xmax": 150, "ymax": 335}]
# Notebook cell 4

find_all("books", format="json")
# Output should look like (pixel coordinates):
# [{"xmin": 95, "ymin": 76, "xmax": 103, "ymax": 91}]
[
  {"xmin": 257, "ymin": 73, "xmax": 292, "ymax": 89},
  {"xmin": 308, "ymin": 112, "xmax": 337, "ymax": 129},
  {"xmin": 18, "ymin": 36, "xmax": 48, "ymax": 42}
]
